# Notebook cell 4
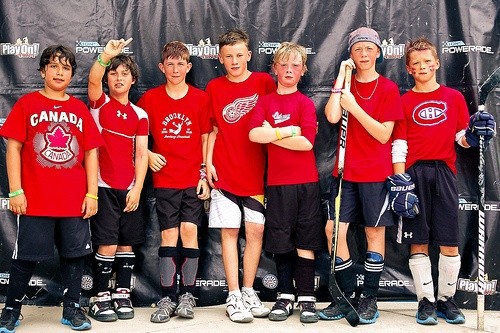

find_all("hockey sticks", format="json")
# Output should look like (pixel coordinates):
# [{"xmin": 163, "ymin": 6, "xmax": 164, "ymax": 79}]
[
  {"xmin": 328, "ymin": 66, "xmax": 360, "ymax": 328},
  {"xmin": 477, "ymin": 66, "xmax": 500, "ymax": 330}
]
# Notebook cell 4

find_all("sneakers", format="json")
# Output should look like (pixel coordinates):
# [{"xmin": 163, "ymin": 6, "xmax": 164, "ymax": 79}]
[
  {"xmin": 268, "ymin": 293, "xmax": 296, "ymax": 321},
  {"xmin": 149, "ymin": 296, "xmax": 177, "ymax": 322},
  {"xmin": 0, "ymin": 306, "xmax": 24, "ymax": 333},
  {"xmin": 224, "ymin": 294, "xmax": 255, "ymax": 323},
  {"xmin": 111, "ymin": 288, "xmax": 134, "ymax": 319},
  {"xmin": 415, "ymin": 296, "xmax": 438, "ymax": 325},
  {"xmin": 316, "ymin": 291, "xmax": 359, "ymax": 320},
  {"xmin": 436, "ymin": 295, "xmax": 466, "ymax": 323},
  {"xmin": 60, "ymin": 301, "xmax": 92, "ymax": 330},
  {"xmin": 296, "ymin": 296, "xmax": 318, "ymax": 323},
  {"xmin": 355, "ymin": 294, "xmax": 380, "ymax": 324},
  {"xmin": 174, "ymin": 292, "xmax": 198, "ymax": 319},
  {"xmin": 87, "ymin": 290, "xmax": 118, "ymax": 321},
  {"xmin": 240, "ymin": 288, "xmax": 271, "ymax": 316}
]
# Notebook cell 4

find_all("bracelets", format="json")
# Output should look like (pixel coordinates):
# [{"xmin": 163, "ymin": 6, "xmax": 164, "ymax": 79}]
[
  {"xmin": 201, "ymin": 163, "xmax": 206, "ymax": 167},
  {"xmin": 291, "ymin": 126, "xmax": 297, "ymax": 136},
  {"xmin": 86, "ymin": 193, "xmax": 98, "ymax": 200},
  {"xmin": 276, "ymin": 128, "xmax": 283, "ymax": 140},
  {"xmin": 98, "ymin": 54, "xmax": 111, "ymax": 67},
  {"xmin": 9, "ymin": 189, "xmax": 24, "ymax": 198},
  {"xmin": 331, "ymin": 88, "xmax": 342, "ymax": 93},
  {"xmin": 199, "ymin": 170, "xmax": 207, "ymax": 182}
]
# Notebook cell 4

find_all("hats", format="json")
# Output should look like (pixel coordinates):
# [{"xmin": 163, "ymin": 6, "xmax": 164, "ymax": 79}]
[{"xmin": 347, "ymin": 26, "xmax": 384, "ymax": 64}]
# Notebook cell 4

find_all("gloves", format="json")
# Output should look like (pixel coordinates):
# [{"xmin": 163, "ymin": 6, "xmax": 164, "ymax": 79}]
[
  {"xmin": 464, "ymin": 110, "xmax": 497, "ymax": 153},
  {"xmin": 385, "ymin": 173, "xmax": 420, "ymax": 218}
]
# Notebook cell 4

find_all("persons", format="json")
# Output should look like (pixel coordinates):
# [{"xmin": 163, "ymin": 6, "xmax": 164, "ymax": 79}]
[
  {"xmin": 88, "ymin": 37, "xmax": 149, "ymax": 321},
  {"xmin": 206, "ymin": 29, "xmax": 278, "ymax": 322},
  {"xmin": 136, "ymin": 41, "xmax": 215, "ymax": 322},
  {"xmin": 392, "ymin": 38, "xmax": 497, "ymax": 324},
  {"xmin": 0, "ymin": 44, "xmax": 103, "ymax": 333},
  {"xmin": 317, "ymin": 27, "xmax": 405, "ymax": 323},
  {"xmin": 248, "ymin": 42, "xmax": 320, "ymax": 320}
]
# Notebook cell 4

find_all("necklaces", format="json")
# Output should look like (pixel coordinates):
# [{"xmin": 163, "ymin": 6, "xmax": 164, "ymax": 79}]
[{"xmin": 354, "ymin": 77, "xmax": 378, "ymax": 100}]
